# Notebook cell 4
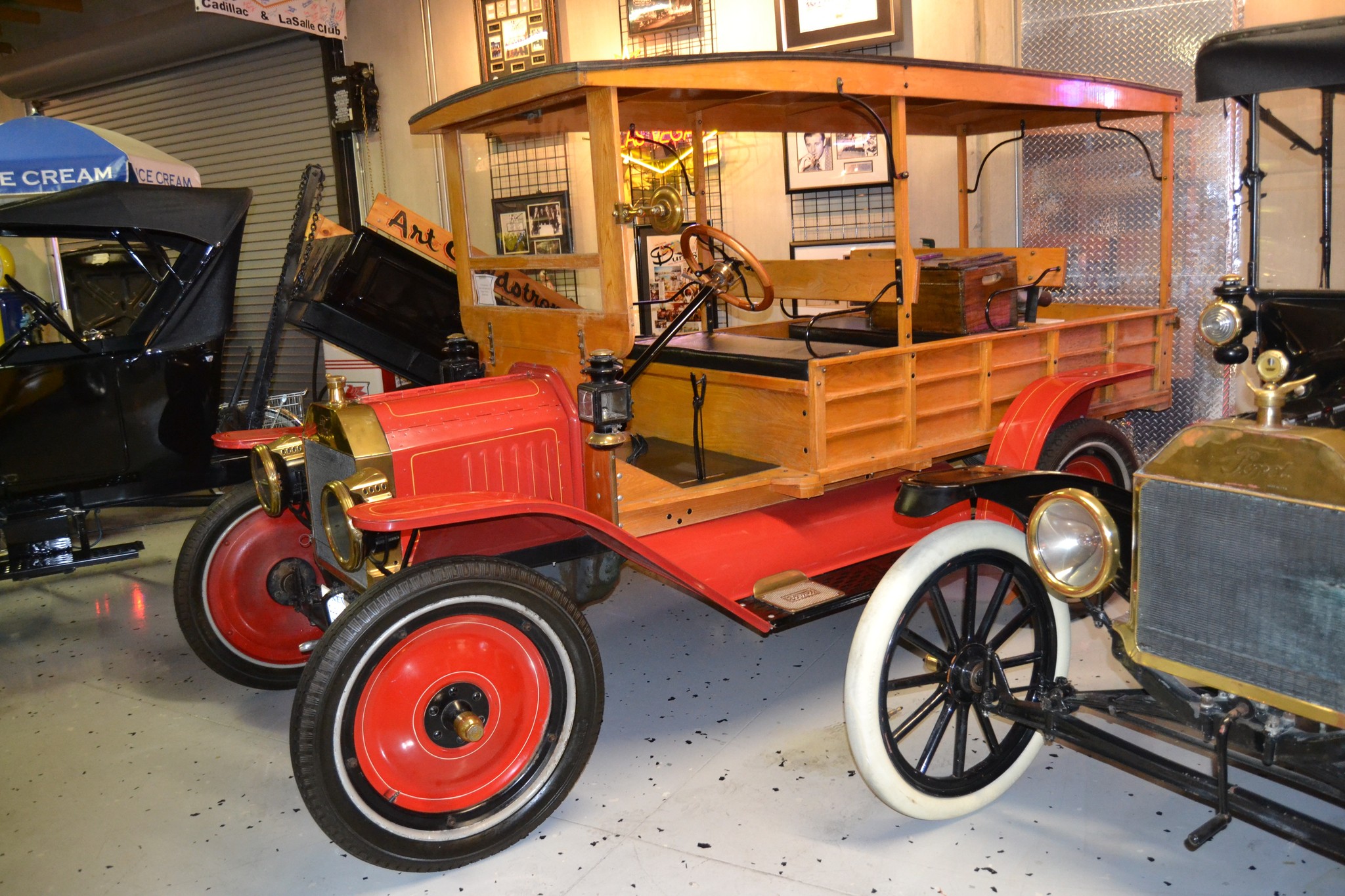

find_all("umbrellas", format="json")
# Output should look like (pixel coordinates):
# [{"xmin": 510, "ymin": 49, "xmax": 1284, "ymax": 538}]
[{"xmin": 0, "ymin": 108, "xmax": 203, "ymax": 344}]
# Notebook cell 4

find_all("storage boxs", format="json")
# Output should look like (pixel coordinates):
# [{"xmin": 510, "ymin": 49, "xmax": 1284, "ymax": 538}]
[{"xmin": 865, "ymin": 250, "xmax": 1018, "ymax": 337}]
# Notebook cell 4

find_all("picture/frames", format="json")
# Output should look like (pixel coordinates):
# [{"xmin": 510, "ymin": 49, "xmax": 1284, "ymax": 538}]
[
  {"xmin": 491, "ymin": 189, "xmax": 575, "ymax": 256},
  {"xmin": 782, "ymin": 131, "xmax": 892, "ymax": 195},
  {"xmin": 625, "ymin": 0, "xmax": 701, "ymax": 38},
  {"xmin": 789, "ymin": 235, "xmax": 897, "ymax": 320},
  {"xmin": 472, "ymin": 0, "xmax": 560, "ymax": 85},
  {"xmin": 774, "ymin": 0, "xmax": 905, "ymax": 54},
  {"xmin": 632, "ymin": 219, "xmax": 719, "ymax": 339}
]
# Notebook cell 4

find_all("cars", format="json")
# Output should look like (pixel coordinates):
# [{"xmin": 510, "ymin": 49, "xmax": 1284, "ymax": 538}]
[{"xmin": 1, "ymin": 19, "xmax": 1345, "ymax": 873}]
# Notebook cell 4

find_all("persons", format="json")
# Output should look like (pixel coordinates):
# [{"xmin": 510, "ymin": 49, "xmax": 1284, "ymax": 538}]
[
  {"xmin": 797, "ymin": 132, "xmax": 833, "ymax": 173},
  {"xmin": 530, "ymin": 204, "xmax": 561, "ymax": 235}
]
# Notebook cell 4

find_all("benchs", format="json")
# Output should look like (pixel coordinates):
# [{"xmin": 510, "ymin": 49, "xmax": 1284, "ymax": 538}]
[
  {"xmin": 619, "ymin": 256, "xmax": 924, "ymax": 385},
  {"xmin": 787, "ymin": 246, "xmax": 1068, "ymax": 348}
]
